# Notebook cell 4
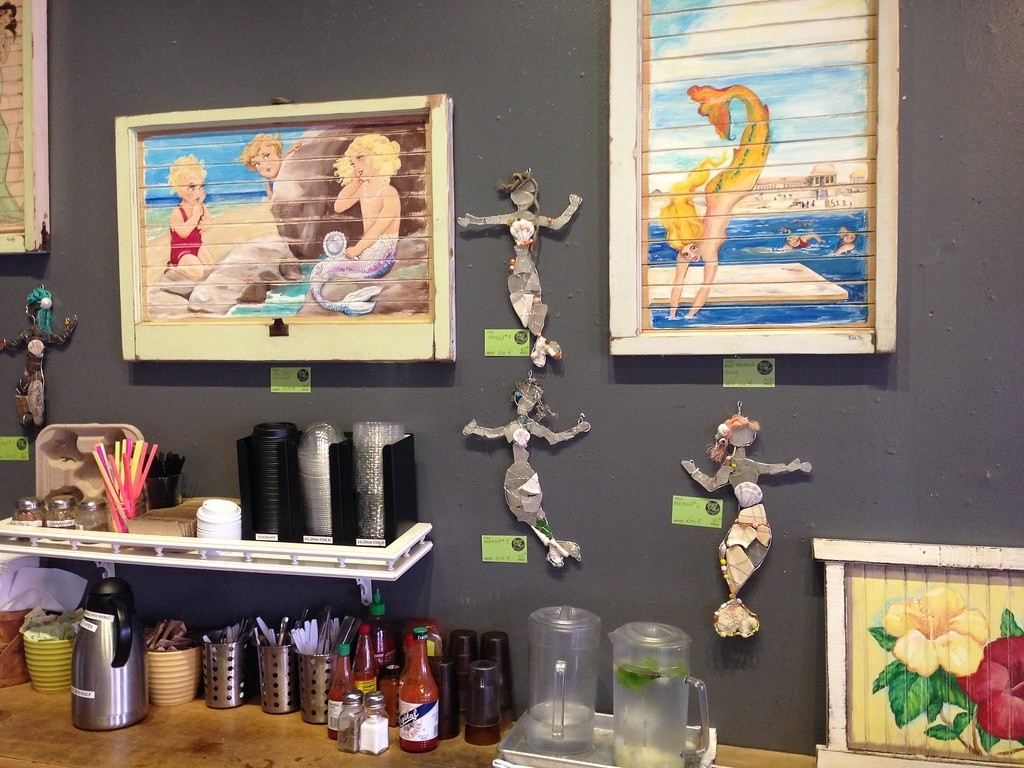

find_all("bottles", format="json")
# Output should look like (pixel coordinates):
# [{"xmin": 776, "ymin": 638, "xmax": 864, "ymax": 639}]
[
  {"xmin": 327, "ymin": 589, "xmax": 440, "ymax": 756},
  {"xmin": 12, "ymin": 494, "xmax": 109, "ymax": 544}
]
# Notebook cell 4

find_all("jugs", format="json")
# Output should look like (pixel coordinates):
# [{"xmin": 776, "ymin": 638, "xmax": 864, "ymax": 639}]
[
  {"xmin": 526, "ymin": 604, "xmax": 602, "ymax": 758},
  {"xmin": 71, "ymin": 577, "xmax": 149, "ymax": 731},
  {"xmin": 608, "ymin": 621, "xmax": 710, "ymax": 768}
]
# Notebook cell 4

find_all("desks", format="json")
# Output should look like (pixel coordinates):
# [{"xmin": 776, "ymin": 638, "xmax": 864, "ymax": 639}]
[{"xmin": 0, "ymin": 679, "xmax": 811, "ymax": 768}]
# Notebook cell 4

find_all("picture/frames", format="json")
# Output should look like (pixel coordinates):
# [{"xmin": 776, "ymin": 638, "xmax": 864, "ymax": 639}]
[
  {"xmin": 0, "ymin": 0, "xmax": 53, "ymax": 255},
  {"xmin": 814, "ymin": 539, "xmax": 1024, "ymax": 767},
  {"xmin": 607, "ymin": 0, "xmax": 904, "ymax": 356},
  {"xmin": 114, "ymin": 94, "xmax": 462, "ymax": 368}
]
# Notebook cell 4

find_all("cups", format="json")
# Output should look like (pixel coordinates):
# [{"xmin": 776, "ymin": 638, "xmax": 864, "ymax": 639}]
[
  {"xmin": 296, "ymin": 419, "xmax": 348, "ymax": 537},
  {"xmin": 23, "ymin": 628, "xmax": 76, "ymax": 694},
  {"xmin": 104, "ymin": 493, "xmax": 147, "ymax": 548},
  {"xmin": 146, "ymin": 645, "xmax": 202, "ymax": 707},
  {"xmin": 353, "ymin": 421, "xmax": 406, "ymax": 539},
  {"xmin": 243, "ymin": 421, "xmax": 300, "ymax": 543},
  {"xmin": 143, "ymin": 472, "xmax": 183, "ymax": 511},
  {"xmin": 196, "ymin": 499, "xmax": 241, "ymax": 541},
  {"xmin": 429, "ymin": 628, "xmax": 513, "ymax": 746}
]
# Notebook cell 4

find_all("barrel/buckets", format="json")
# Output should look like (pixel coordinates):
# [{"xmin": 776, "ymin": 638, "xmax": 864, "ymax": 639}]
[{"xmin": 0, "ymin": 609, "xmax": 32, "ymax": 687}]
